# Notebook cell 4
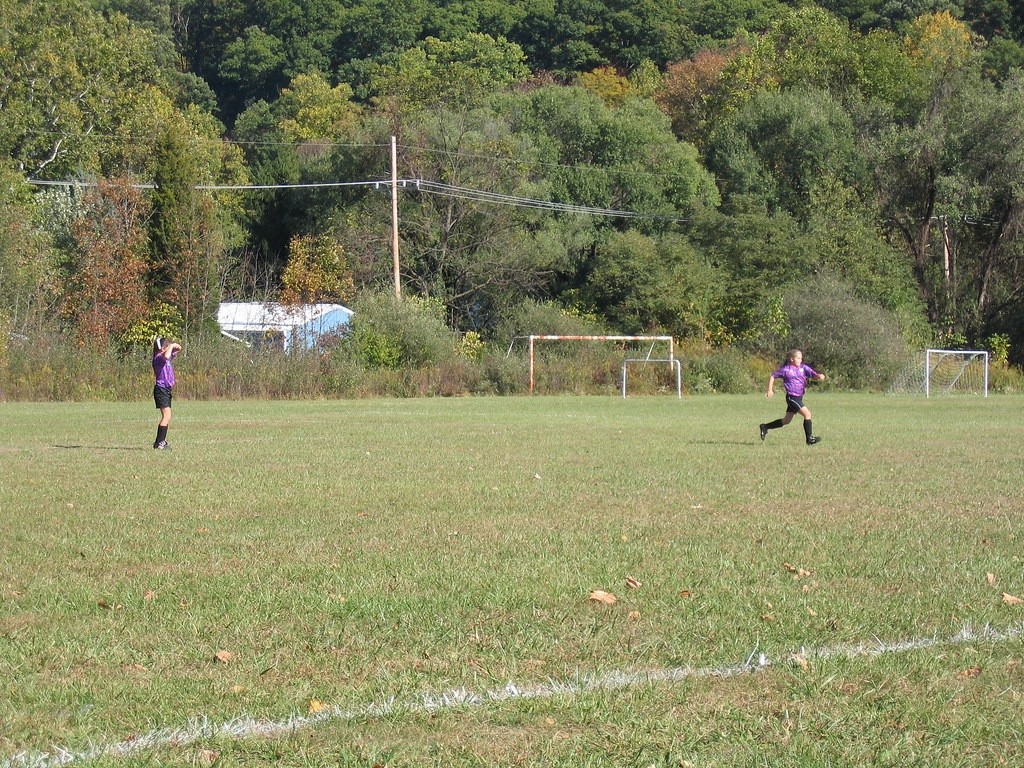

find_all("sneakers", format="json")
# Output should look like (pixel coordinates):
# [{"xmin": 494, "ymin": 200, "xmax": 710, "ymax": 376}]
[
  {"xmin": 760, "ymin": 424, "xmax": 769, "ymax": 440},
  {"xmin": 806, "ymin": 436, "xmax": 822, "ymax": 445},
  {"xmin": 153, "ymin": 441, "xmax": 169, "ymax": 452}
]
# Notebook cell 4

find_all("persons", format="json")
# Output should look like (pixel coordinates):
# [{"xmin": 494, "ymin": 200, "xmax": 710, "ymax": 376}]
[
  {"xmin": 152, "ymin": 337, "xmax": 182, "ymax": 451},
  {"xmin": 759, "ymin": 348, "xmax": 825, "ymax": 445}
]
